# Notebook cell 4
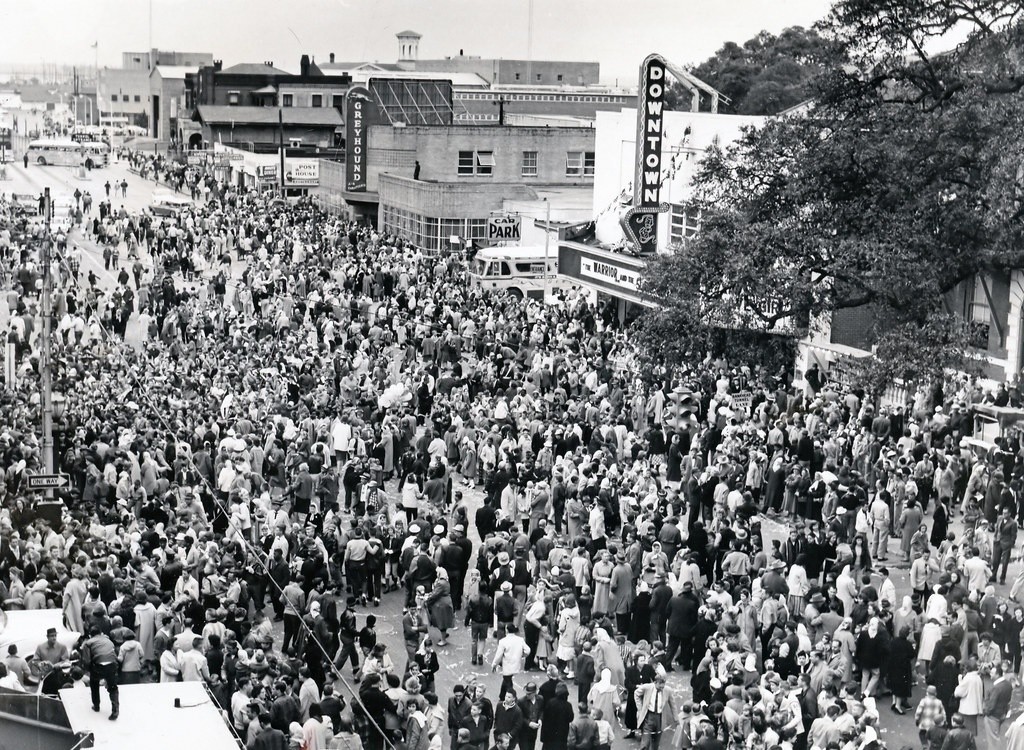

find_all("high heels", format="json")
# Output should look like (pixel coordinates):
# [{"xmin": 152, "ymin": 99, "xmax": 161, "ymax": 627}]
[{"xmin": 890, "ymin": 704, "xmax": 906, "ymax": 715}]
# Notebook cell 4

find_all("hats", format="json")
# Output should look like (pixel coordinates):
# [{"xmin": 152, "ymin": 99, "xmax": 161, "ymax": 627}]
[
  {"xmin": 836, "ymin": 506, "xmax": 847, "ymax": 514},
  {"xmin": 47, "ymin": 628, "xmax": 58, "ymax": 637},
  {"xmin": 498, "ymin": 552, "xmax": 509, "ymax": 566},
  {"xmin": 736, "ymin": 529, "xmax": 748, "ymax": 539},
  {"xmin": 516, "ymin": 546, "xmax": 526, "ymax": 555},
  {"xmin": 409, "ymin": 524, "xmax": 421, "ymax": 533},
  {"xmin": 434, "ymin": 524, "xmax": 444, "ymax": 534},
  {"xmin": 710, "ymin": 678, "xmax": 721, "ymax": 689},
  {"xmin": 524, "ymin": 681, "xmax": 539, "ymax": 694},
  {"xmin": 452, "ymin": 524, "xmax": 464, "ymax": 531},
  {"xmin": 771, "ymin": 560, "xmax": 786, "ymax": 569},
  {"xmin": 614, "ymin": 553, "xmax": 627, "ymax": 563},
  {"xmin": 810, "ymin": 592, "xmax": 826, "ymax": 603},
  {"xmin": 500, "ymin": 581, "xmax": 512, "ymax": 591}
]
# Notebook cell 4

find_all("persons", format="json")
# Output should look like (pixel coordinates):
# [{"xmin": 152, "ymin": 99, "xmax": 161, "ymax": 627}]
[
  {"xmin": 413, "ymin": 160, "xmax": 420, "ymax": 180},
  {"xmin": 0, "ymin": 115, "xmax": 1023, "ymax": 749}
]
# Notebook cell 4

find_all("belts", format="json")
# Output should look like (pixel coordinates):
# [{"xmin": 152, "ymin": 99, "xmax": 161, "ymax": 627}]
[{"xmin": 648, "ymin": 710, "xmax": 662, "ymax": 715}]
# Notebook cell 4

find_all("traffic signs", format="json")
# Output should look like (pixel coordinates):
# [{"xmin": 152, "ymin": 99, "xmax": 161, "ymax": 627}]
[{"xmin": 27, "ymin": 474, "xmax": 70, "ymax": 489}]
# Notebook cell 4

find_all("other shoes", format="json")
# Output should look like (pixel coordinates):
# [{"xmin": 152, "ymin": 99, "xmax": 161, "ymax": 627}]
[
  {"xmin": 624, "ymin": 731, "xmax": 635, "ymax": 739},
  {"xmin": 1010, "ymin": 594, "xmax": 1020, "ymax": 604},
  {"xmin": 108, "ymin": 711, "xmax": 120, "ymax": 720},
  {"xmin": 92, "ymin": 705, "xmax": 100, "ymax": 713}
]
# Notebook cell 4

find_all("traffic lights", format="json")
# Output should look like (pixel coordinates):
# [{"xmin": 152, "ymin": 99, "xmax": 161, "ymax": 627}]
[
  {"xmin": 665, "ymin": 393, "xmax": 678, "ymax": 433},
  {"xmin": 679, "ymin": 392, "xmax": 692, "ymax": 434}
]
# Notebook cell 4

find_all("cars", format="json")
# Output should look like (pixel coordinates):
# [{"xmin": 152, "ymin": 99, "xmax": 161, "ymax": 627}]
[
  {"xmin": 70, "ymin": 124, "xmax": 147, "ymax": 136},
  {"xmin": 149, "ymin": 198, "xmax": 192, "ymax": 218}
]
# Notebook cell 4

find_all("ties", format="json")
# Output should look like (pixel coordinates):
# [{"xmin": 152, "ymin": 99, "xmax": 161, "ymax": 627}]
[{"xmin": 655, "ymin": 690, "xmax": 659, "ymax": 715}]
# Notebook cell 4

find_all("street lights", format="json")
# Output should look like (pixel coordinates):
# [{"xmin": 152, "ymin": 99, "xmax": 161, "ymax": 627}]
[
  {"xmin": 543, "ymin": 197, "xmax": 550, "ymax": 282},
  {"xmin": 51, "ymin": 91, "xmax": 63, "ymax": 135},
  {"xmin": 79, "ymin": 94, "xmax": 92, "ymax": 134},
  {"xmin": 64, "ymin": 92, "xmax": 77, "ymax": 134}
]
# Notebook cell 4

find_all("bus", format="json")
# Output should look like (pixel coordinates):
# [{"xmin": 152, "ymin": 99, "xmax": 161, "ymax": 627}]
[
  {"xmin": 472, "ymin": 246, "xmax": 558, "ymax": 304},
  {"xmin": 26, "ymin": 140, "xmax": 107, "ymax": 168}
]
